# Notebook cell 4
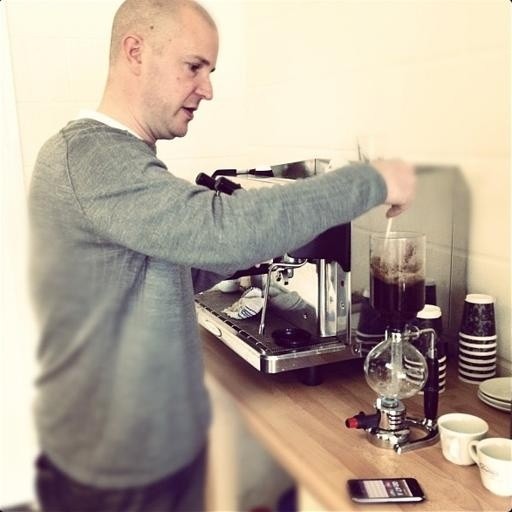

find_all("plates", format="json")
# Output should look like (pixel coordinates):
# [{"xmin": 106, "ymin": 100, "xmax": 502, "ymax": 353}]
[{"xmin": 476, "ymin": 377, "xmax": 512, "ymax": 413}]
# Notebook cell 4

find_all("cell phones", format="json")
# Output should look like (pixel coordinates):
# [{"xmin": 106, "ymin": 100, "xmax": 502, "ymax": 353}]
[{"xmin": 347, "ymin": 478, "xmax": 425, "ymax": 503}]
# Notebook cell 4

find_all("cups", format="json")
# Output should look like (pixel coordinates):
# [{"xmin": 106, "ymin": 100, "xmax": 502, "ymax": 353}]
[
  {"xmin": 356, "ymin": 291, "xmax": 385, "ymax": 356},
  {"xmin": 439, "ymin": 412, "xmax": 489, "ymax": 467},
  {"xmin": 402, "ymin": 279, "xmax": 447, "ymax": 394},
  {"xmin": 455, "ymin": 294, "xmax": 496, "ymax": 384},
  {"xmin": 467, "ymin": 438, "xmax": 511, "ymax": 498},
  {"xmin": 368, "ymin": 230, "xmax": 427, "ymax": 335}
]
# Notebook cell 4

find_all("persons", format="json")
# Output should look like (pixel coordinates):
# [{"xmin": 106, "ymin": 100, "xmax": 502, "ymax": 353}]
[{"xmin": 26, "ymin": 0, "xmax": 416, "ymax": 512}]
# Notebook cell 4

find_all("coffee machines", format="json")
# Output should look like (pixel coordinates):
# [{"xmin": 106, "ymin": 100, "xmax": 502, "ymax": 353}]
[{"xmin": 193, "ymin": 167, "xmax": 454, "ymax": 376}]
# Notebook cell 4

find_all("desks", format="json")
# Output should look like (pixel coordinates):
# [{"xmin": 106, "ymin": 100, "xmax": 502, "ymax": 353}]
[{"xmin": 195, "ymin": 322, "xmax": 512, "ymax": 512}]
